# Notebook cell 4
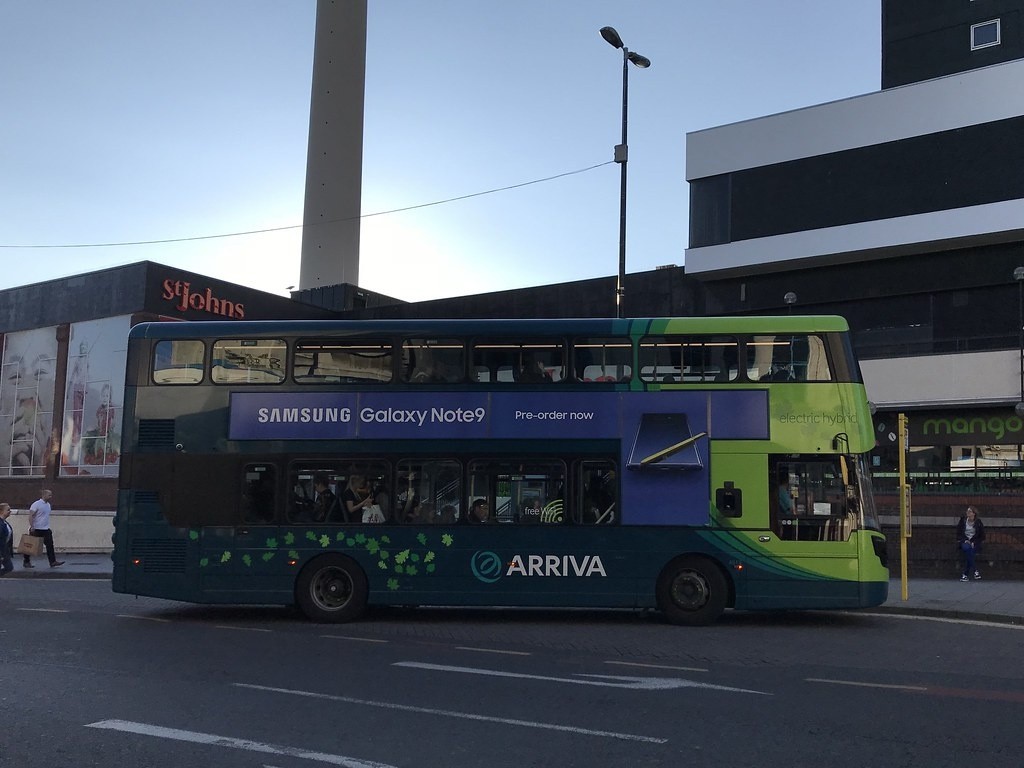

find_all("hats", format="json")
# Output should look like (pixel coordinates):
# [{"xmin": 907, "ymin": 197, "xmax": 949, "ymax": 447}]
[{"xmin": 473, "ymin": 499, "xmax": 486, "ymax": 508}]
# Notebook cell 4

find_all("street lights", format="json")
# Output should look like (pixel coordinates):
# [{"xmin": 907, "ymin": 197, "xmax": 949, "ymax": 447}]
[{"xmin": 599, "ymin": 25, "xmax": 651, "ymax": 376}]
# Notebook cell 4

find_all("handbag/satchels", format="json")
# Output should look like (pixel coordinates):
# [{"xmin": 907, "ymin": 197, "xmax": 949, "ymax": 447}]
[
  {"xmin": 362, "ymin": 504, "xmax": 386, "ymax": 524},
  {"xmin": 17, "ymin": 531, "xmax": 44, "ymax": 556}
]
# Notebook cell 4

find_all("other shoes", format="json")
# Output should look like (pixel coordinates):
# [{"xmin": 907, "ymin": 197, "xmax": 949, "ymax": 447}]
[
  {"xmin": 973, "ymin": 570, "xmax": 981, "ymax": 579},
  {"xmin": 960, "ymin": 573, "xmax": 969, "ymax": 582},
  {"xmin": 24, "ymin": 563, "xmax": 35, "ymax": 568},
  {"xmin": 51, "ymin": 560, "xmax": 66, "ymax": 568}
]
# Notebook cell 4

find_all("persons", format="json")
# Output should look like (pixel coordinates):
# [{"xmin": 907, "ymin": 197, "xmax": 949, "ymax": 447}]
[
  {"xmin": 414, "ymin": 356, "xmax": 795, "ymax": 382},
  {"xmin": 0, "ymin": 330, "xmax": 116, "ymax": 475},
  {"xmin": 289, "ymin": 465, "xmax": 615, "ymax": 527},
  {"xmin": 23, "ymin": 489, "xmax": 65, "ymax": 568},
  {"xmin": 0, "ymin": 503, "xmax": 14, "ymax": 576},
  {"xmin": 771, "ymin": 471, "xmax": 798, "ymax": 538},
  {"xmin": 957, "ymin": 505, "xmax": 986, "ymax": 581}
]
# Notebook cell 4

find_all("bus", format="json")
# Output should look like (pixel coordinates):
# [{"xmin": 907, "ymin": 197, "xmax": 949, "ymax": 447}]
[{"xmin": 112, "ymin": 315, "xmax": 893, "ymax": 626}]
